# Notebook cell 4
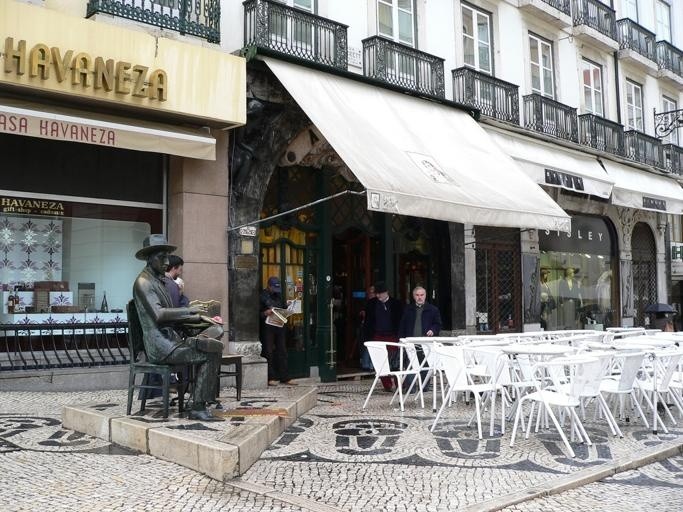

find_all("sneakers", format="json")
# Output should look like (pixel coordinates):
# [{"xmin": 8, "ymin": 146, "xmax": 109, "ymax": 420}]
[
  {"xmin": 285, "ymin": 379, "xmax": 298, "ymax": 385},
  {"xmin": 268, "ymin": 379, "xmax": 278, "ymax": 386}
]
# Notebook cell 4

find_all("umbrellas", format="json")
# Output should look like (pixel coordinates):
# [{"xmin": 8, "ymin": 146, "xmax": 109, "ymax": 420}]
[{"xmin": 641, "ymin": 303, "xmax": 677, "ymax": 314}]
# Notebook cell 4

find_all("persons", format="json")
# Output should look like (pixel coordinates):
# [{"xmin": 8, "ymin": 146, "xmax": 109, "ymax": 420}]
[
  {"xmin": 137, "ymin": 256, "xmax": 186, "ymax": 400},
  {"xmin": 398, "ymin": 286, "xmax": 442, "ymax": 394},
  {"xmin": 595, "ymin": 270, "xmax": 613, "ymax": 328},
  {"xmin": 541, "ymin": 268, "xmax": 552, "ymax": 331},
  {"xmin": 132, "ymin": 233, "xmax": 227, "ymax": 422},
  {"xmin": 361, "ymin": 285, "xmax": 377, "ymax": 372},
  {"xmin": 259, "ymin": 276, "xmax": 293, "ymax": 387},
  {"xmin": 558, "ymin": 268, "xmax": 579, "ymax": 324},
  {"xmin": 576, "ymin": 271, "xmax": 588, "ymax": 317},
  {"xmin": 173, "ymin": 276, "xmax": 190, "ymax": 306},
  {"xmin": 650, "ymin": 312, "xmax": 668, "ymax": 322},
  {"xmin": 360, "ymin": 284, "xmax": 405, "ymax": 391}
]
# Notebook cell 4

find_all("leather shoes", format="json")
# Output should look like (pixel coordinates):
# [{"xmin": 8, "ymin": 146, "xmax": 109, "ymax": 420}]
[{"xmin": 190, "ymin": 410, "xmax": 225, "ymax": 421}]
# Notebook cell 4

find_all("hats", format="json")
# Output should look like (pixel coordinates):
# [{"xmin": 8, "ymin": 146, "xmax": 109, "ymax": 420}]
[
  {"xmin": 135, "ymin": 233, "xmax": 178, "ymax": 260},
  {"xmin": 269, "ymin": 276, "xmax": 282, "ymax": 293},
  {"xmin": 373, "ymin": 280, "xmax": 388, "ymax": 293}
]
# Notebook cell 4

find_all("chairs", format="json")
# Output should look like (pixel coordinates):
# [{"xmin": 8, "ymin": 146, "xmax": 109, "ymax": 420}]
[
  {"xmin": 469, "ymin": 326, "xmax": 661, "ymax": 348},
  {"xmin": 125, "ymin": 297, "xmax": 187, "ymax": 419},
  {"xmin": 508, "ymin": 358, "xmax": 600, "ymax": 458},
  {"xmin": 579, "ymin": 351, "xmax": 650, "ymax": 437},
  {"xmin": 389, "ymin": 336, "xmax": 469, "ymax": 407},
  {"xmin": 467, "ymin": 348, "xmax": 541, "ymax": 433},
  {"xmin": 544, "ymin": 351, "xmax": 625, "ymax": 439},
  {"xmin": 363, "ymin": 341, "xmax": 434, "ymax": 412},
  {"xmin": 429, "ymin": 345, "xmax": 509, "ymax": 440},
  {"xmin": 188, "ymin": 300, "xmax": 244, "ymax": 401},
  {"xmin": 619, "ymin": 352, "xmax": 683, "ymax": 435}
]
